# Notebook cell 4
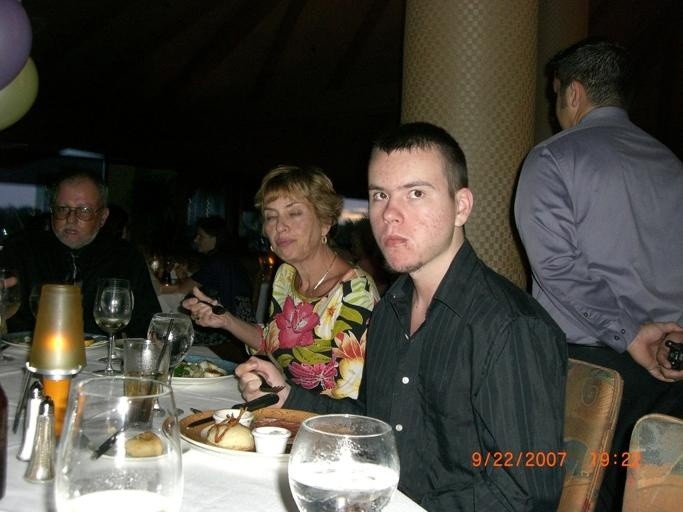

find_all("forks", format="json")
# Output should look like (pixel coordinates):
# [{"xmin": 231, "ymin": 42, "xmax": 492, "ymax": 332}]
[{"xmin": 255, "ymin": 375, "xmax": 287, "ymax": 393}]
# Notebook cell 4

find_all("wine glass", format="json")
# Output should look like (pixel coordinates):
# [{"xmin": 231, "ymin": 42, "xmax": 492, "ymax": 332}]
[
  {"xmin": 144, "ymin": 310, "xmax": 195, "ymax": 420},
  {"xmin": 92, "ymin": 278, "xmax": 135, "ymax": 376}
]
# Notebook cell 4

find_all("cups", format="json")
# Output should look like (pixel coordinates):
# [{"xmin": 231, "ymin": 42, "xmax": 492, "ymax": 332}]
[
  {"xmin": 286, "ymin": 415, "xmax": 401, "ymax": 511},
  {"xmin": 52, "ymin": 377, "xmax": 185, "ymax": 510},
  {"xmin": 251, "ymin": 426, "xmax": 292, "ymax": 453},
  {"xmin": 211, "ymin": 408, "xmax": 255, "ymax": 430},
  {"xmin": 122, "ymin": 337, "xmax": 169, "ymax": 422}
]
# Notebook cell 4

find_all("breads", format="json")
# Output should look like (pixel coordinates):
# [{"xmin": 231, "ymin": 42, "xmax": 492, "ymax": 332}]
[
  {"xmin": 126, "ymin": 430, "xmax": 163, "ymax": 457},
  {"xmin": 199, "ymin": 409, "xmax": 254, "ymax": 451}
]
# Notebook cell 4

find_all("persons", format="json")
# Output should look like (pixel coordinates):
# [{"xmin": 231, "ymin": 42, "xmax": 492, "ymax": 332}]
[
  {"xmin": 100, "ymin": 202, "xmax": 381, "ymax": 347},
  {"xmin": 0, "ymin": 161, "xmax": 162, "ymax": 340},
  {"xmin": 178, "ymin": 160, "xmax": 381, "ymax": 400},
  {"xmin": 230, "ymin": 120, "xmax": 570, "ymax": 510},
  {"xmin": 509, "ymin": 33, "xmax": 683, "ymax": 510}
]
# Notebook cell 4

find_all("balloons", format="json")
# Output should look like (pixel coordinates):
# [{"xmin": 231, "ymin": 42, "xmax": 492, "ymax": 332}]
[
  {"xmin": 0, "ymin": 0, "xmax": 32, "ymax": 90},
  {"xmin": 0, "ymin": 59, "xmax": 40, "ymax": 132}
]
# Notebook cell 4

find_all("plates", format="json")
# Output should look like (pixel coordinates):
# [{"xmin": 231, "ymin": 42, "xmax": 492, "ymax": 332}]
[
  {"xmin": 73, "ymin": 428, "xmax": 179, "ymax": 463},
  {"xmin": 2, "ymin": 327, "xmax": 112, "ymax": 350},
  {"xmin": 179, "ymin": 404, "xmax": 360, "ymax": 453},
  {"xmin": 167, "ymin": 353, "xmax": 239, "ymax": 383}
]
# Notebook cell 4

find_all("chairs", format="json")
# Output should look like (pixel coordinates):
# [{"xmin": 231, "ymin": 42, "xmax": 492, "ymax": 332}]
[
  {"xmin": 556, "ymin": 357, "xmax": 623, "ymax": 512},
  {"xmin": 621, "ymin": 414, "xmax": 683, "ymax": 512}
]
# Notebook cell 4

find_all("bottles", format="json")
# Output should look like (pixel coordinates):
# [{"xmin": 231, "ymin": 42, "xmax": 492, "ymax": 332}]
[{"xmin": 17, "ymin": 383, "xmax": 55, "ymax": 487}]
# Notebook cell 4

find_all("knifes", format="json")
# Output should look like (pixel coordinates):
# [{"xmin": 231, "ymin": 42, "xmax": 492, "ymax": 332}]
[{"xmin": 187, "ymin": 393, "xmax": 281, "ymax": 429}]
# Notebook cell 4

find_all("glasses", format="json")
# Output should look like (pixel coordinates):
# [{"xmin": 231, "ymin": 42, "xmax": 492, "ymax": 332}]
[{"xmin": 52, "ymin": 205, "xmax": 104, "ymax": 221}]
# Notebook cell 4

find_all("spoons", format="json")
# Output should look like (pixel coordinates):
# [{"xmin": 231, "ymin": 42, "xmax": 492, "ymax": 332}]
[{"xmin": 188, "ymin": 295, "xmax": 228, "ymax": 317}]
